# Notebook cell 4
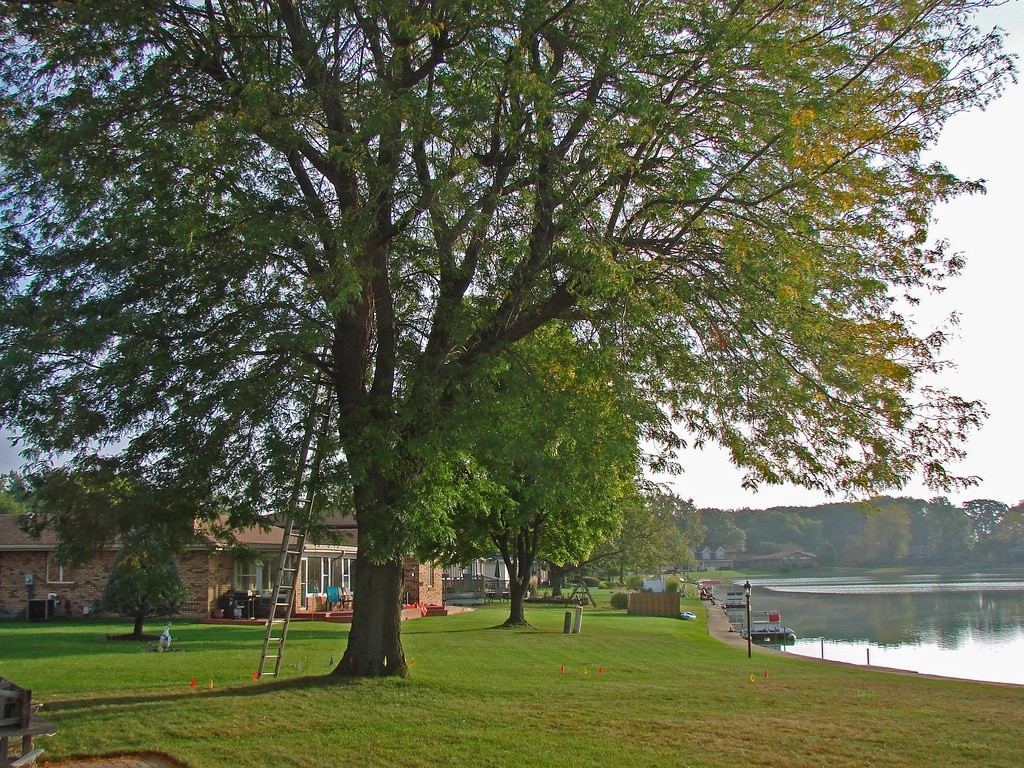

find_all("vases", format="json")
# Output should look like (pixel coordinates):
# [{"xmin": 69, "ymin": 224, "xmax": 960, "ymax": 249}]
[{"xmin": 212, "ymin": 609, "xmax": 224, "ymax": 619}]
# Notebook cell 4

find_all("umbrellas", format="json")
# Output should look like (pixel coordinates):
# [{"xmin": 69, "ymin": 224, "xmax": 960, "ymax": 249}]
[{"xmin": 494, "ymin": 560, "xmax": 500, "ymax": 580}]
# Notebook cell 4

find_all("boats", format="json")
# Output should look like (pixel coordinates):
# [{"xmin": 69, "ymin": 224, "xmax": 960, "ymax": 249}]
[
  {"xmin": 739, "ymin": 610, "xmax": 795, "ymax": 641},
  {"xmin": 721, "ymin": 591, "xmax": 751, "ymax": 611}
]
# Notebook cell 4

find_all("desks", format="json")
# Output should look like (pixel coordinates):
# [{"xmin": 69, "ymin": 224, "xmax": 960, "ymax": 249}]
[
  {"xmin": 486, "ymin": 592, "xmax": 495, "ymax": 603},
  {"xmin": 341, "ymin": 594, "xmax": 354, "ymax": 609}
]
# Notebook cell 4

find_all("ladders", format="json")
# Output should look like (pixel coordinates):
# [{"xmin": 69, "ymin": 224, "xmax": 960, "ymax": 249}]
[{"xmin": 257, "ymin": 331, "xmax": 336, "ymax": 680}]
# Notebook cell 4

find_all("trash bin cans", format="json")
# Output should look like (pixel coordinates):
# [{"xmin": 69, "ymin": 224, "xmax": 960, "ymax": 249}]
[{"xmin": 233, "ymin": 589, "xmax": 252, "ymax": 620}]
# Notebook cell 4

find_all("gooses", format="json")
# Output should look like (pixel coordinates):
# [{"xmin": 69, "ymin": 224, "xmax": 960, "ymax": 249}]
[
  {"xmin": 158, "ymin": 624, "xmax": 173, "ymax": 648},
  {"xmin": 330, "ymin": 656, "xmax": 333, "ymax": 665}
]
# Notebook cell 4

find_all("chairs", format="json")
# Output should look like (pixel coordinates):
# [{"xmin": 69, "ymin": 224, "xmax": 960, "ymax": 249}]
[
  {"xmin": 326, "ymin": 586, "xmax": 344, "ymax": 610},
  {"xmin": 499, "ymin": 588, "xmax": 511, "ymax": 603}
]
